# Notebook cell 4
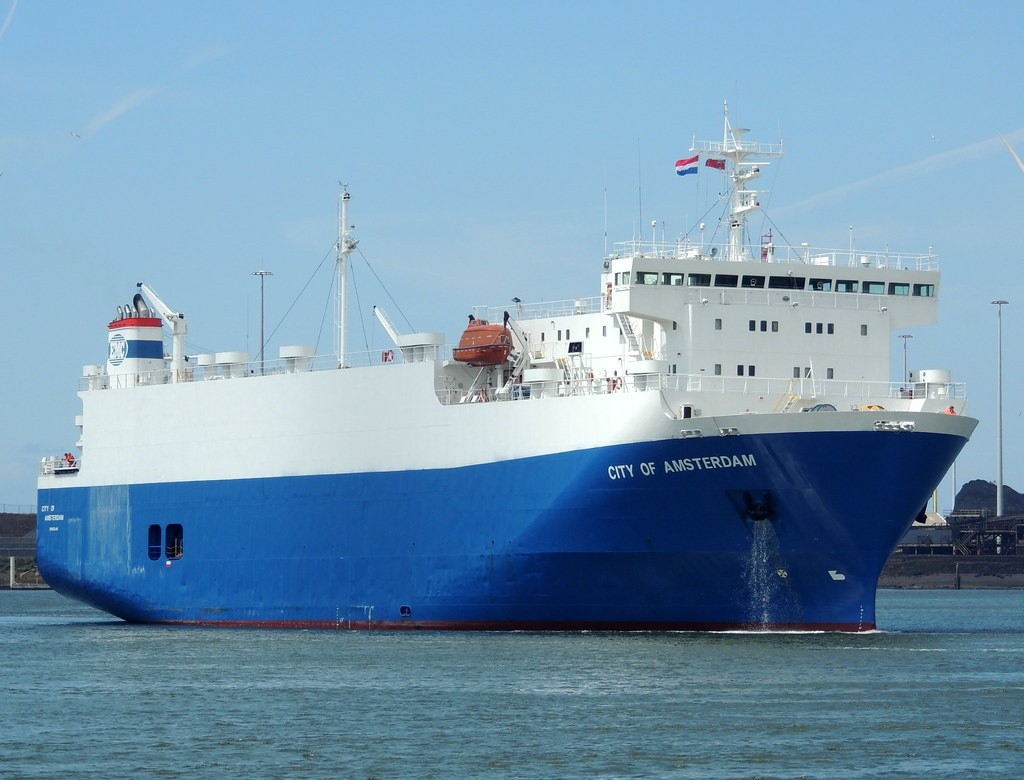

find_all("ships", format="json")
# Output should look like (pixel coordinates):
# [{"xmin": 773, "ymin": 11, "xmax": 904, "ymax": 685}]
[{"xmin": 35, "ymin": 101, "xmax": 982, "ymax": 636}]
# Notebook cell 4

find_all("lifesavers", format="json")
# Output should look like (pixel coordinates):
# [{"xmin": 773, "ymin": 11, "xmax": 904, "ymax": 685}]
[{"xmin": 386, "ymin": 350, "xmax": 393, "ymax": 363}]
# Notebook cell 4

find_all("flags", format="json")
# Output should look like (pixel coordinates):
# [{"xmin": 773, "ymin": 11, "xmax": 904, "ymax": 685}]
[
  {"xmin": 705, "ymin": 158, "xmax": 725, "ymax": 170},
  {"xmin": 675, "ymin": 154, "xmax": 699, "ymax": 176}
]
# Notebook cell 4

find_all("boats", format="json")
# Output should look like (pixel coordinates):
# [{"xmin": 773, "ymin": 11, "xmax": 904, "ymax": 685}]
[{"xmin": 453, "ymin": 324, "xmax": 512, "ymax": 365}]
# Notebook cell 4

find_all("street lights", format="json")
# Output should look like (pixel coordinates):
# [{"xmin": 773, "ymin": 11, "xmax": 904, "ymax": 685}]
[
  {"xmin": 991, "ymin": 299, "xmax": 1010, "ymax": 555},
  {"xmin": 251, "ymin": 270, "xmax": 273, "ymax": 375},
  {"xmin": 898, "ymin": 334, "xmax": 913, "ymax": 389}
]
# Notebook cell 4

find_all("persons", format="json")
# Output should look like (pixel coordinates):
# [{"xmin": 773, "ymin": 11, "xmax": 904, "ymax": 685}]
[
  {"xmin": 946, "ymin": 406, "xmax": 955, "ymax": 415},
  {"xmin": 64, "ymin": 453, "xmax": 77, "ymax": 467}
]
